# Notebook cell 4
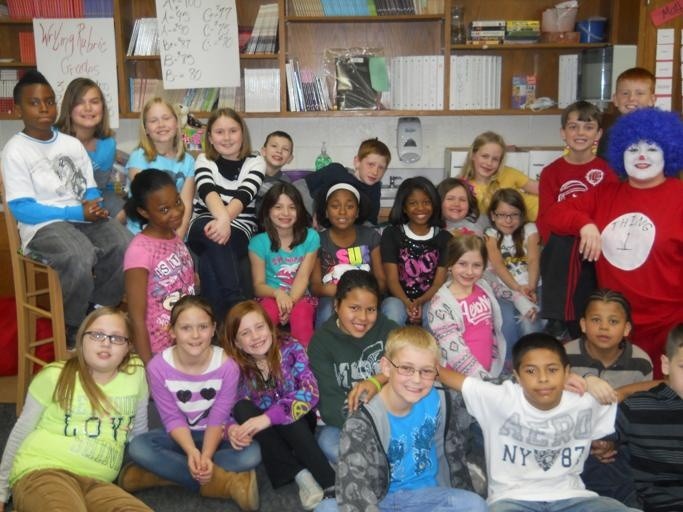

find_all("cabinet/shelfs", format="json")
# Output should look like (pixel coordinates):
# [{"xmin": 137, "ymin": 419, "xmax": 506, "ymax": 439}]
[{"xmin": 0, "ymin": 0, "xmax": 681, "ymax": 122}]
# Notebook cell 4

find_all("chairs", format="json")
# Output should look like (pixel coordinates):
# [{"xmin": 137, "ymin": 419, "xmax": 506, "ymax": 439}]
[{"xmin": 1, "ymin": 200, "xmax": 80, "ymax": 426}]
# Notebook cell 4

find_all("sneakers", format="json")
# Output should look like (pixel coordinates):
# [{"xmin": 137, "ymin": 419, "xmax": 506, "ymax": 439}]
[{"xmin": 299, "ymin": 475, "xmax": 323, "ymax": 509}]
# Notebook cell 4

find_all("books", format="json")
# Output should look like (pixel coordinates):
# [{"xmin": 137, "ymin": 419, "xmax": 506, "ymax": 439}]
[
  {"xmin": 564, "ymin": 54, "xmax": 573, "ymax": 108},
  {"xmin": 1, "ymin": 0, "xmax": 116, "ymax": 111},
  {"xmin": 286, "ymin": 0, "xmax": 445, "ymax": 113},
  {"xmin": 571, "ymin": 54, "xmax": 580, "ymax": 103},
  {"xmin": 449, "ymin": 7, "xmax": 543, "ymax": 111},
  {"xmin": 558, "ymin": 55, "xmax": 566, "ymax": 109},
  {"xmin": 127, "ymin": 4, "xmax": 280, "ymax": 111}
]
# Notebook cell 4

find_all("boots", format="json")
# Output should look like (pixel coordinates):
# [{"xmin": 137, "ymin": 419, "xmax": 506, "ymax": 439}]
[
  {"xmin": 119, "ymin": 462, "xmax": 170, "ymax": 492},
  {"xmin": 200, "ymin": 464, "xmax": 259, "ymax": 511}
]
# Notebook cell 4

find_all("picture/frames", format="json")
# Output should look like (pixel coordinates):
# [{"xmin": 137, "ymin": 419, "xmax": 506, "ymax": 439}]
[{"xmin": 445, "ymin": 145, "xmax": 574, "ymax": 181}]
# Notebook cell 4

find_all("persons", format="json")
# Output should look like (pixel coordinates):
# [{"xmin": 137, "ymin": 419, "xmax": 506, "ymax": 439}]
[{"xmin": 1, "ymin": 67, "xmax": 683, "ymax": 511}]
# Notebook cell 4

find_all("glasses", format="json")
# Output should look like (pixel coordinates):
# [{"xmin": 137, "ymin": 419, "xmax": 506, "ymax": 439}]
[
  {"xmin": 494, "ymin": 212, "xmax": 519, "ymax": 220},
  {"xmin": 83, "ymin": 331, "xmax": 127, "ymax": 345},
  {"xmin": 385, "ymin": 355, "xmax": 437, "ymax": 378}
]
